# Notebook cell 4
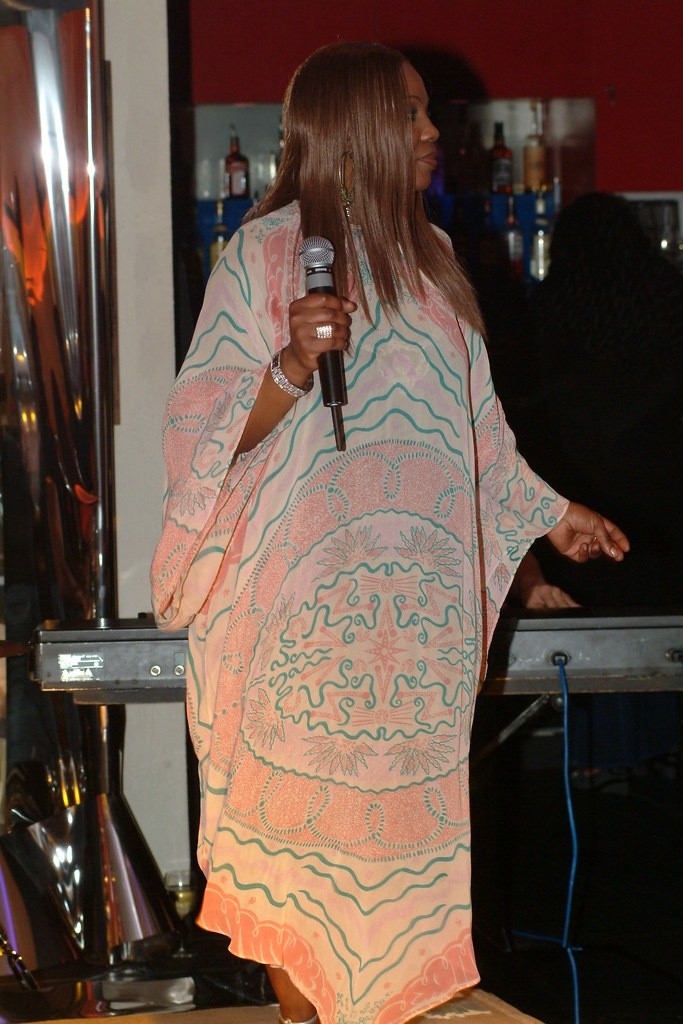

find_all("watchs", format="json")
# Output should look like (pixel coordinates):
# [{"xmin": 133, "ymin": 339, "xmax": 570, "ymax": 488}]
[{"xmin": 270, "ymin": 348, "xmax": 314, "ymax": 398}]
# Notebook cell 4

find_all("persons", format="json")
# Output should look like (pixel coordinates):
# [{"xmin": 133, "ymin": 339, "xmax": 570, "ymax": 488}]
[
  {"xmin": 149, "ymin": 41, "xmax": 631, "ymax": 1023},
  {"xmin": 515, "ymin": 549, "xmax": 580, "ymax": 609}
]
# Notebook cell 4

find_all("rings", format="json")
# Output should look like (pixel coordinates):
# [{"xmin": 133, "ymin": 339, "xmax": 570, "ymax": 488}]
[
  {"xmin": 593, "ymin": 536, "xmax": 597, "ymax": 543},
  {"xmin": 316, "ymin": 322, "xmax": 332, "ymax": 339}
]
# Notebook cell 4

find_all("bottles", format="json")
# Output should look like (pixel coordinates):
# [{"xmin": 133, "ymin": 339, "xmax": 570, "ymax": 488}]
[
  {"xmin": 531, "ymin": 193, "xmax": 553, "ymax": 283},
  {"xmin": 185, "ymin": 198, "xmax": 232, "ymax": 282},
  {"xmin": 428, "ymin": 193, "xmax": 500, "ymax": 278},
  {"xmin": 524, "ymin": 101, "xmax": 548, "ymax": 192},
  {"xmin": 223, "ymin": 129, "xmax": 251, "ymax": 200},
  {"xmin": 500, "ymin": 196, "xmax": 528, "ymax": 279},
  {"xmin": 489, "ymin": 123, "xmax": 513, "ymax": 193},
  {"xmin": 448, "ymin": 105, "xmax": 478, "ymax": 191}
]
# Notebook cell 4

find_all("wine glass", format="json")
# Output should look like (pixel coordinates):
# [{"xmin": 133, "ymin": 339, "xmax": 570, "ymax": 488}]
[{"xmin": 162, "ymin": 868, "xmax": 200, "ymax": 962}]
